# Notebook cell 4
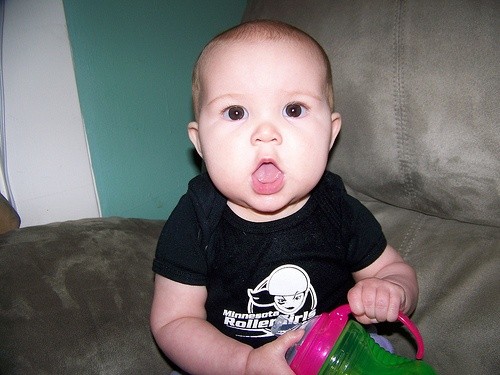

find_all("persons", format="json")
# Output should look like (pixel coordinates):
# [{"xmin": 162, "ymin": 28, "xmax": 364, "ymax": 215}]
[{"xmin": 151, "ymin": 21, "xmax": 418, "ymax": 374}]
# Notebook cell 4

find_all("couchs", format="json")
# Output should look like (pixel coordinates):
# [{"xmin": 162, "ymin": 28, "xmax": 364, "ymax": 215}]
[{"xmin": 0, "ymin": 0, "xmax": 500, "ymax": 375}]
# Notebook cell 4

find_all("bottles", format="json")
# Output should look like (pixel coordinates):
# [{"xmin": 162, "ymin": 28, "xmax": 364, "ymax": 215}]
[{"xmin": 271, "ymin": 303, "xmax": 437, "ymax": 375}]
